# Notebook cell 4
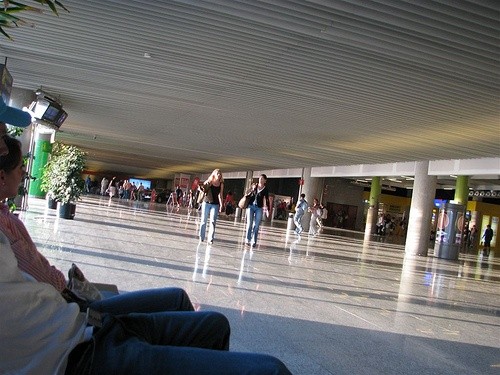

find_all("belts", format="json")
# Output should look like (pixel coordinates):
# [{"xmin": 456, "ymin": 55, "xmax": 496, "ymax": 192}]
[{"xmin": 64, "ymin": 307, "xmax": 104, "ymax": 375}]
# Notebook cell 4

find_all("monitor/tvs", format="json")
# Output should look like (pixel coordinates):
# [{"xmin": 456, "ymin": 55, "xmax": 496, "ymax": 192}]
[
  {"xmin": 33, "ymin": 99, "xmax": 60, "ymax": 120},
  {"xmin": 53, "ymin": 110, "xmax": 68, "ymax": 127}
]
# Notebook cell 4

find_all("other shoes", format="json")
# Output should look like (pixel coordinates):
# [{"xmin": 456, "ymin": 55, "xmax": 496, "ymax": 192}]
[
  {"xmin": 298, "ymin": 228, "xmax": 304, "ymax": 234},
  {"xmin": 200, "ymin": 237, "xmax": 205, "ymax": 242},
  {"xmin": 245, "ymin": 239, "xmax": 250, "ymax": 246},
  {"xmin": 251, "ymin": 243, "xmax": 256, "ymax": 248},
  {"xmin": 208, "ymin": 240, "xmax": 213, "ymax": 244}
]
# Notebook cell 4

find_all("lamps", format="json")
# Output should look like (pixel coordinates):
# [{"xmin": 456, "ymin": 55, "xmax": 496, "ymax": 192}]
[
  {"xmin": 468, "ymin": 190, "xmax": 474, "ymax": 197},
  {"xmin": 490, "ymin": 191, "xmax": 497, "ymax": 198},
  {"xmin": 474, "ymin": 191, "xmax": 479, "ymax": 197},
  {"xmin": 485, "ymin": 191, "xmax": 491, "ymax": 197},
  {"xmin": 497, "ymin": 191, "xmax": 500, "ymax": 198},
  {"xmin": 480, "ymin": 191, "xmax": 485, "ymax": 197}
]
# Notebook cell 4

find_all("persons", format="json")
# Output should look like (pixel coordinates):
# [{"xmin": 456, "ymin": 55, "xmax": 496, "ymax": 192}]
[
  {"xmin": 0, "ymin": 136, "xmax": 230, "ymax": 351},
  {"xmin": 197, "ymin": 168, "xmax": 224, "ymax": 243},
  {"xmin": 238, "ymin": 174, "xmax": 271, "ymax": 247},
  {"xmin": 277, "ymin": 194, "xmax": 328, "ymax": 235},
  {"xmin": 85, "ymin": 173, "xmax": 236, "ymax": 217},
  {"xmin": 0, "ymin": 92, "xmax": 292, "ymax": 375},
  {"xmin": 376, "ymin": 211, "xmax": 494, "ymax": 257}
]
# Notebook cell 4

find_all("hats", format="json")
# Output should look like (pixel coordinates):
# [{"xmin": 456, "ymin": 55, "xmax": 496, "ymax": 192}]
[{"xmin": 0, "ymin": 95, "xmax": 31, "ymax": 127}]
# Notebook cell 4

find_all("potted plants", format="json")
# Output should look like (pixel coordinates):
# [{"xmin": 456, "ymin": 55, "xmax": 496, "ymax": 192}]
[{"xmin": 37, "ymin": 139, "xmax": 89, "ymax": 221}]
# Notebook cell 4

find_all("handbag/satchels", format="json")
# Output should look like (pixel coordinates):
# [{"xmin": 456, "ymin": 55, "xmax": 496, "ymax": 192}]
[
  {"xmin": 197, "ymin": 180, "xmax": 213, "ymax": 204},
  {"xmin": 237, "ymin": 183, "xmax": 257, "ymax": 209}
]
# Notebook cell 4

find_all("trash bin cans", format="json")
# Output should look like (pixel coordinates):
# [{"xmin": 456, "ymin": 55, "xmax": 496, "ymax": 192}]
[
  {"xmin": 287, "ymin": 213, "xmax": 295, "ymax": 231},
  {"xmin": 235, "ymin": 208, "xmax": 241, "ymax": 218}
]
